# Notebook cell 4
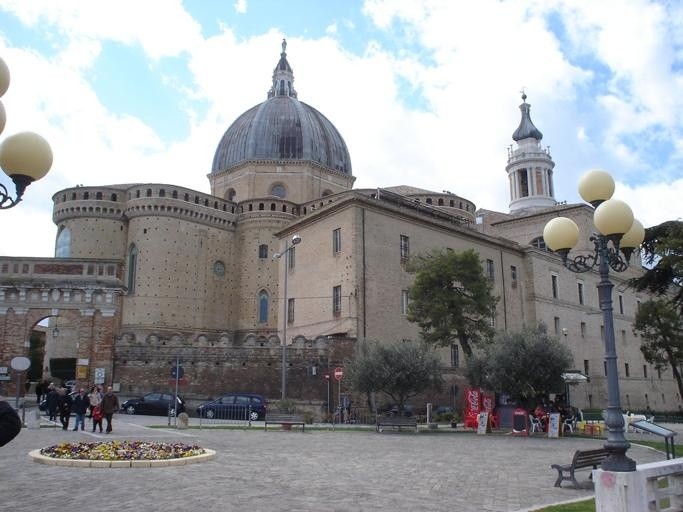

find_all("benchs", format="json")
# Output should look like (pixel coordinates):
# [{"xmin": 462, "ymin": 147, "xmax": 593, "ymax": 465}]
[
  {"xmin": 376, "ymin": 414, "xmax": 418, "ymax": 433},
  {"xmin": 550, "ymin": 447, "xmax": 610, "ymax": 489},
  {"xmin": 264, "ymin": 412, "xmax": 306, "ymax": 432}
]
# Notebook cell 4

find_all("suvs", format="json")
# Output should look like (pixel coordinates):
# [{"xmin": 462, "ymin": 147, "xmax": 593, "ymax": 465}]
[
  {"xmin": 120, "ymin": 392, "xmax": 185, "ymax": 417},
  {"xmin": 196, "ymin": 393, "xmax": 267, "ymax": 421}
]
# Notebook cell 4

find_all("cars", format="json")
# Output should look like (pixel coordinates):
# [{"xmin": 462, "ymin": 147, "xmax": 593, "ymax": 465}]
[
  {"xmin": 377, "ymin": 402, "xmax": 413, "ymax": 418},
  {"xmin": 435, "ymin": 406, "xmax": 454, "ymax": 416}
]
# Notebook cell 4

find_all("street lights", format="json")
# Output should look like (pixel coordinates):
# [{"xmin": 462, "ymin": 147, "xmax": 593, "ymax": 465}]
[
  {"xmin": 272, "ymin": 235, "xmax": 301, "ymax": 401},
  {"xmin": 542, "ymin": 168, "xmax": 645, "ymax": 472}
]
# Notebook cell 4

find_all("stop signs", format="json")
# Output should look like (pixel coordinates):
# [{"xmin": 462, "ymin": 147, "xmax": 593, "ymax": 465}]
[
  {"xmin": 334, "ymin": 367, "xmax": 344, "ymax": 381},
  {"xmin": 324, "ymin": 373, "xmax": 331, "ymax": 382}
]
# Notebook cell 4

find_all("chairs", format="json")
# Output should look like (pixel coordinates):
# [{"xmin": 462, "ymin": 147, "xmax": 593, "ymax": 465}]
[
  {"xmin": 528, "ymin": 413, "xmax": 544, "ymax": 433},
  {"xmin": 621, "ymin": 411, "xmax": 655, "ymax": 436},
  {"xmin": 561, "ymin": 414, "xmax": 577, "ymax": 435}
]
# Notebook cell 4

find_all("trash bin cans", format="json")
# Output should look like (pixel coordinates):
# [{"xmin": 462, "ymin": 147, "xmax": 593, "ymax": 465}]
[
  {"xmin": 25, "ymin": 406, "xmax": 40, "ymax": 429},
  {"xmin": 178, "ymin": 412, "xmax": 189, "ymax": 429}
]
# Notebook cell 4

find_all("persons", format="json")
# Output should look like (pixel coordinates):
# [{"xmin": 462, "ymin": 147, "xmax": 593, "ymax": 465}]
[
  {"xmin": 22, "ymin": 377, "xmax": 120, "ymax": 435},
  {"xmin": 506, "ymin": 393, "xmax": 576, "ymax": 434}
]
